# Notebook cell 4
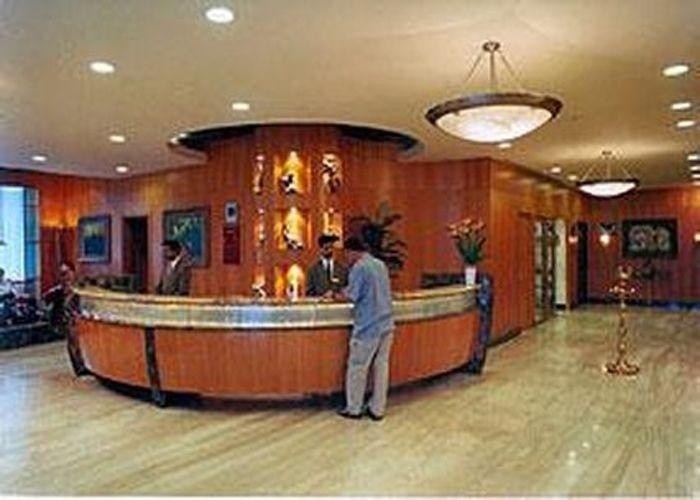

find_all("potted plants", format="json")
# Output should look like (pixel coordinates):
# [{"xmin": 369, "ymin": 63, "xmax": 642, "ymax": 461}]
[{"xmin": 448, "ymin": 218, "xmax": 488, "ymax": 286}]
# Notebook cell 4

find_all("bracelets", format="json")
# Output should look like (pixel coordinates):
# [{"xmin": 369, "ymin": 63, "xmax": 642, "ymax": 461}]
[{"xmin": 332, "ymin": 292, "xmax": 336, "ymax": 299}]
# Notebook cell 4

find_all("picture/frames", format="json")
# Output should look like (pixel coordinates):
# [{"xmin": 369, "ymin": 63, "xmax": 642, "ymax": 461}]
[
  {"xmin": 76, "ymin": 213, "xmax": 112, "ymax": 264},
  {"xmin": 162, "ymin": 207, "xmax": 212, "ymax": 268}
]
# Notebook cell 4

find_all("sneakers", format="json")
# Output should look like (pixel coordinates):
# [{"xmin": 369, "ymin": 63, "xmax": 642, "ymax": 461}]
[{"xmin": 338, "ymin": 408, "xmax": 383, "ymax": 421}]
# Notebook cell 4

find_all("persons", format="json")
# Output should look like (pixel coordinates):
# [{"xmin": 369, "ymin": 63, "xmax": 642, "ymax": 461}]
[
  {"xmin": 323, "ymin": 236, "xmax": 395, "ymax": 421},
  {"xmin": 306, "ymin": 235, "xmax": 349, "ymax": 298},
  {"xmin": 154, "ymin": 239, "xmax": 195, "ymax": 297}
]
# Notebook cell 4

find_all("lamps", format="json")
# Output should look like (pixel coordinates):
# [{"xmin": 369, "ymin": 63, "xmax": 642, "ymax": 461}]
[
  {"xmin": 576, "ymin": 149, "xmax": 640, "ymax": 199},
  {"xmin": 425, "ymin": 39, "xmax": 563, "ymax": 146}
]
